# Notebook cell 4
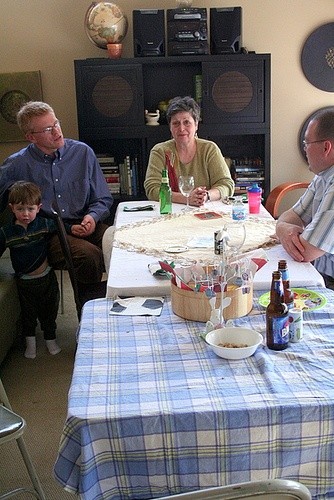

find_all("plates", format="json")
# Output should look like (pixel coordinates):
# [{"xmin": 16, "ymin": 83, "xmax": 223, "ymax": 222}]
[
  {"xmin": 166, "ymin": 247, "xmax": 188, "ymax": 253},
  {"xmin": 259, "ymin": 288, "xmax": 328, "ymax": 313}
]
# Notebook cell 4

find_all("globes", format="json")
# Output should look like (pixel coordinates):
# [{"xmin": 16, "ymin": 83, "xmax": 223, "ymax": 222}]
[{"xmin": 84, "ymin": 2, "xmax": 128, "ymax": 50}]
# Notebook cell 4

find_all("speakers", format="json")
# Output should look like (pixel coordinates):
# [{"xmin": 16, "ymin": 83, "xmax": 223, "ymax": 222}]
[
  {"xmin": 208, "ymin": 6, "xmax": 241, "ymax": 54},
  {"xmin": 133, "ymin": 9, "xmax": 165, "ymax": 56}
]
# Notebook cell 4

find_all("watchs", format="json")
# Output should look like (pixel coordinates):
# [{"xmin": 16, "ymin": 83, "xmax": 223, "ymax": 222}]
[{"xmin": 206, "ymin": 191, "xmax": 212, "ymax": 201}]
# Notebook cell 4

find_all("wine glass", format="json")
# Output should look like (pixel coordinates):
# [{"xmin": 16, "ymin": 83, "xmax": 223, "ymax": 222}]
[
  {"xmin": 178, "ymin": 176, "xmax": 194, "ymax": 211},
  {"xmin": 223, "ymin": 220, "xmax": 245, "ymax": 253}
]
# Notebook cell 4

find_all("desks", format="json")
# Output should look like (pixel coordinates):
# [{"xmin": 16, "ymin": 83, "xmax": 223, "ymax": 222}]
[
  {"xmin": 54, "ymin": 299, "xmax": 334, "ymax": 500},
  {"xmin": 106, "ymin": 201, "xmax": 326, "ymax": 298}
]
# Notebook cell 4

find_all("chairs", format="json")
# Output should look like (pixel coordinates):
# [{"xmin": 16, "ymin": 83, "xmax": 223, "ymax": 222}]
[
  {"xmin": 37, "ymin": 211, "xmax": 83, "ymax": 323},
  {"xmin": 0, "ymin": 378, "xmax": 47, "ymax": 500}
]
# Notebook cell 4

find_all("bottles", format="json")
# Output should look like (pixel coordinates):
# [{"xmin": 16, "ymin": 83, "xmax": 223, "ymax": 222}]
[
  {"xmin": 233, "ymin": 199, "xmax": 248, "ymax": 220},
  {"xmin": 266, "ymin": 260, "xmax": 294, "ymax": 350},
  {"xmin": 159, "ymin": 169, "xmax": 171, "ymax": 214},
  {"xmin": 247, "ymin": 182, "xmax": 263, "ymax": 214}
]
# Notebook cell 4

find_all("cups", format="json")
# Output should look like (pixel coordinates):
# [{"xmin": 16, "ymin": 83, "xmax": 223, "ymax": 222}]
[{"xmin": 107, "ymin": 44, "xmax": 122, "ymax": 58}]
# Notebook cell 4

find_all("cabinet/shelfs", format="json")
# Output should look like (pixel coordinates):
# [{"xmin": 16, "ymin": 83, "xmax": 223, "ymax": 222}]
[{"xmin": 74, "ymin": 54, "xmax": 271, "ymax": 208}]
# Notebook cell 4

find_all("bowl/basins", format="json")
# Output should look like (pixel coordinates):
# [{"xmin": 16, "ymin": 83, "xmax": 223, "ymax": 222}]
[{"xmin": 204, "ymin": 327, "xmax": 263, "ymax": 360}]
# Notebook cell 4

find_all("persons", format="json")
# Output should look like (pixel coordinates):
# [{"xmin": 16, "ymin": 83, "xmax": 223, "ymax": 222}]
[
  {"xmin": 276, "ymin": 109, "xmax": 334, "ymax": 289},
  {"xmin": 0, "ymin": 102, "xmax": 112, "ymax": 358},
  {"xmin": 144, "ymin": 97, "xmax": 236, "ymax": 208}
]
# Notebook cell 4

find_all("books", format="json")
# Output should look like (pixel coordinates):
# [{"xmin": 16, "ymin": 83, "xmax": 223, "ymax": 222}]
[
  {"xmin": 97, "ymin": 153, "xmax": 138, "ymax": 197},
  {"xmin": 224, "ymin": 156, "xmax": 265, "ymax": 195}
]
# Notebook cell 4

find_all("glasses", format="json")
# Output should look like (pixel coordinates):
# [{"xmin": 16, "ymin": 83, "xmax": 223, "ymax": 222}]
[
  {"xmin": 302, "ymin": 138, "xmax": 327, "ymax": 148},
  {"xmin": 29, "ymin": 120, "xmax": 62, "ymax": 136}
]
